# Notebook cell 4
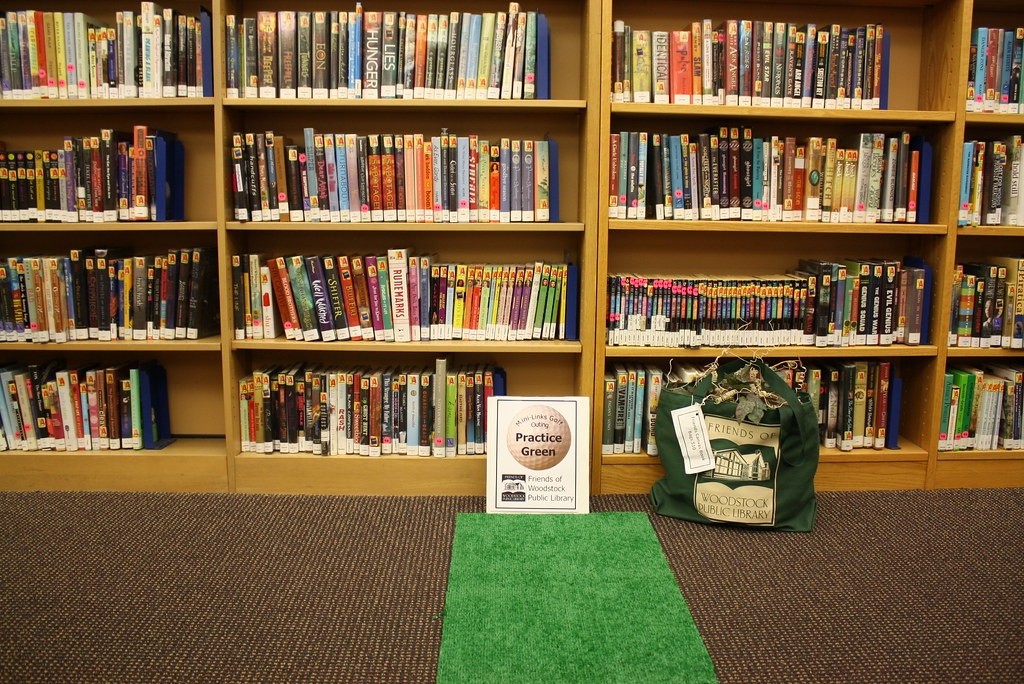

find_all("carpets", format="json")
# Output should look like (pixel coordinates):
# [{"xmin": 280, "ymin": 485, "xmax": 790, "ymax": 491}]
[{"xmin": 435, "ymin": 511, "xmax": 721, "ymax": 684}]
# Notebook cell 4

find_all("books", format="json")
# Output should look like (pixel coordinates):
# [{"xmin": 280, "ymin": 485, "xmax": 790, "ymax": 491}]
[
  {"xmin": 937, "ymin": 29, "xmax": 1021, "ymax": 452},
  {"xmin": 0, "ymin": 0, "xmax": 214, "ymax": 451},
  {"xmin": 224, "ymin": 4, "xmax": 578, "ymax": 459},
  {"xmin": 602, "ymin": 19, "xmax": 929, "ymax": 455}
]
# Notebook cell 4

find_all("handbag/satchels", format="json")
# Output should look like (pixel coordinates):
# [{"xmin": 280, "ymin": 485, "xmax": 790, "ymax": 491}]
[{"xmin": 650, "ymin": 358, "xmax": 819, "ymax": 531}]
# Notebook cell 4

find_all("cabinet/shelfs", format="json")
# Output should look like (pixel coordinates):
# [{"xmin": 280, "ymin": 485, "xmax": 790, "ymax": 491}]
[{"xmin": 0, "ymin": 0, "xmax": 1024, "ymax": 497}]
